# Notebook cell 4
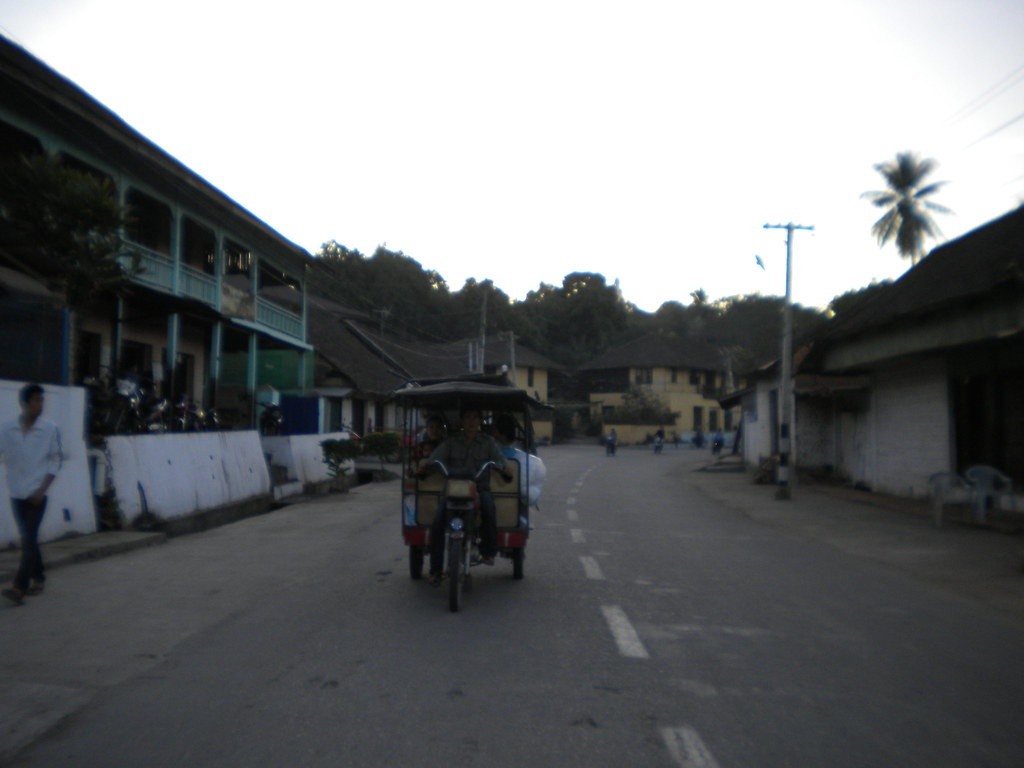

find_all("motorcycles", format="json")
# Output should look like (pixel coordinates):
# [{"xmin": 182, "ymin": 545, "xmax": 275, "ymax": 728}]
[
  {"xmin": 391, "ymin": 379, "xmax": 559, "ymax": 613},
  {"xmin": 259, "ymin": 397, "xmax": 291, "ymax": 433},
  {"xmin": 102, "ymin": 361, "xmax": 231, "ymax": 434}
]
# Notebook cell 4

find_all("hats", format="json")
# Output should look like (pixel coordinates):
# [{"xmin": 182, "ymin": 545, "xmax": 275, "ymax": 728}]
[
  {"xmin": 419, "ymin": 437, "xmax": 437, "ymax": 448},
  {"xmin": 459, "ymin": 400, "xmax": 484, "ymax": 423}
]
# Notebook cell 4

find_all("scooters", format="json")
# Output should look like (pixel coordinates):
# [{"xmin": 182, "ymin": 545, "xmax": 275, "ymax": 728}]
[
  {"xmin": 655, "ymin": 440, "xmax": 665, "ymax": 454},
  {"xmin": 607, "ymin": 437, "xmax": 618, "ymax": 456},
  {"xmin": 712, "ymin": 443, "xmax": 722, "ymax": 455}
]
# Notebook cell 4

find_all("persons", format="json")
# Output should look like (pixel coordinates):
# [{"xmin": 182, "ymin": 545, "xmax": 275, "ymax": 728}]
[
  {"xmin": 608, "ymin": 429, "xmax": 617, "ymax": 454},
  {"xmin": 0, "ymin": 383, "xmax": 63, "ymax": 605},
  {"xmin": 413, "ymin": 406, "xmax": 523, "ymax": 586},
  {"xmin": 655, "ymin": 425, "xmax": 739, "ymax": 455}
]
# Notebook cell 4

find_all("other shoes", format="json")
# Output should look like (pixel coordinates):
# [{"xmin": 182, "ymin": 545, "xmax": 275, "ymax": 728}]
[
  {"xmin": 483, "ymin": 555, "xmax": 496, "ymax": 566},
  {"xmin": 430, "ymin": 572, "xmax": 442, "ymax": 586},
  {"xmin": 1, "ymin": 587, "xmax": 25, "ymax": 605},
  {"xmin": 31, "ymin": 577, "xmax": 46, "ymax": 595}
]
